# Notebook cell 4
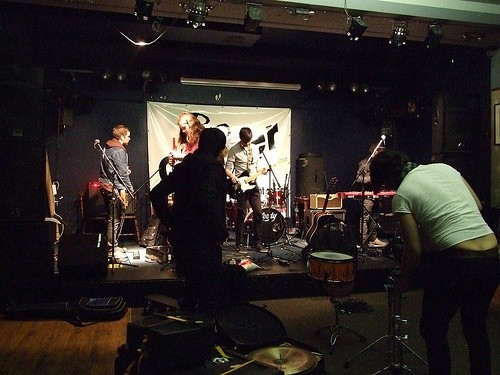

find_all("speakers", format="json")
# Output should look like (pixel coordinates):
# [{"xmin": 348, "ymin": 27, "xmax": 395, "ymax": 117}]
[
  {"xmin": 82, "ymin": 182, "xmax": 135, "ymax": 216},
  {"xmin": 304, "ymin": 194, "xmax": 346, "ymax": 233},
  {"xmin": 56, "ymin": 232, "xmax": 108, "ymax": 281}
]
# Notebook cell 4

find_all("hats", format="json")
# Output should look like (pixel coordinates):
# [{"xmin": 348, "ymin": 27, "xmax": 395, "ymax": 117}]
[{"xmin": 200, "ymin": 128, "xmax": 225, "ymax": 154}]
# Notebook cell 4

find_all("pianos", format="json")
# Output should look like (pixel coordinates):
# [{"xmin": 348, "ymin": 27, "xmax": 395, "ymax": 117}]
[{"xmin": 336, "ymin": 189, "xmax": 397, "ymax": 255}]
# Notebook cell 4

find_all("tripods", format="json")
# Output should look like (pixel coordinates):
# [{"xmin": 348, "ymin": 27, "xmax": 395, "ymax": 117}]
[
  {"xmin": 314, "ymin": 277, "xmax": 430, "ymax": 375},
  {"xmin": 253, "ymin": 152, "xmax": 305, "ymax": 263},
  {"xmin": 99, "ymin": 144, "xmax": 138, "ymax": 274}
]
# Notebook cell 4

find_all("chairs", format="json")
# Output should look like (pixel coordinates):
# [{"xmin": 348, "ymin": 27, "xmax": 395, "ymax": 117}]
[{"xmin": 79, "ymin": 188, "xmax": 140, "ymax": 242}]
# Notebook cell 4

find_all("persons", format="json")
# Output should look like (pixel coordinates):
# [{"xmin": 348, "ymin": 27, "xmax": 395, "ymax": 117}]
[
  {"xmin": 149, "ymin": 128, "xmax": 230, "ymax": 315},
  {"xmin": 225, "ymin": 128, "xmax": 268, "ymax": 253},
  {"xmin": 208, "ymin": 265, "xmax": 286, "ymax": 344},
  {"xmin": 369, "ymin": 149, "xmax": 500, "ymax": 375},
  {"xmin": 99, "ymin": 124, "xmax": 134, "ymax": 256},
  {"xmin": 169, "ymin": 112, "xmax": 205, "ymax": 166},
  {"xmin": 357, "ymin": 142, "xmax": 386, "ymax": 246}
]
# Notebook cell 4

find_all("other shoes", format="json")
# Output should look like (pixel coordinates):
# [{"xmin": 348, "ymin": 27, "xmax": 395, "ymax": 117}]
[
  {"xmin": 255, "ymin": 243, "xmax": 268, "ymax": 253},
  {"xmin": 237, "ymin": 243, "xmax": 247, "ymax": 254}
]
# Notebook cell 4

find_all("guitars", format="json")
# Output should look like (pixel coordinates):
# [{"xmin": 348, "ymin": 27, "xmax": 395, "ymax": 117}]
[
  {"xmin": 226, "ymin": 156, "xmax": 288, "ymax": 200},
  {"xmin": 120, "ymin": 153, "xmax": 129, "ymax": 209},
  {"xmin": 306, "ymin": 177, "xmax": 338, "ymax": 244}
]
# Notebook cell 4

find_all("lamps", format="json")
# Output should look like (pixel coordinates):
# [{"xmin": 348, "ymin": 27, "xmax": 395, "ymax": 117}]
[
  {"xmin": 346, "ymin": 13, "xmax": 368, "ymax": 43},
  {"xmin": 134, "ymin": 0, "xmax": 154, "ymax": 24},
  {"xmin": 180, "ymin": 77, "xmax": 301, "ymax": 91},
  {"xmin": 423, "ymin": 22, "xmax": 443, "ymax": 50},
  {"xmin": 179, "ymin": 0, "xmax": 214, "ymax": 29},
  {"xmin": 389, "ymin": 18, "xmax": 408, "ymax": 47},
  {"xmin": 244, "ymin": 3, "xmax": 264, "ymax": 33}
]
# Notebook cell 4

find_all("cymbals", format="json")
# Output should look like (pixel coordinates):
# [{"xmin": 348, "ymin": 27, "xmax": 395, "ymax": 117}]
[{"xmin": 249, "ymin": 347, "xmax": 318, "ymax": 375}]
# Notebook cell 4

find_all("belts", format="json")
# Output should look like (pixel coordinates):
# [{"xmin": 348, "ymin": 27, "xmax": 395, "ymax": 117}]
[{"xmin": 436, "ymin": 246, "xmax": 498, "ymax": 258}]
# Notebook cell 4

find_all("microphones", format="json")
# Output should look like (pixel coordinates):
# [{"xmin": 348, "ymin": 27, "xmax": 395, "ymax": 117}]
[
  {"xmin": 93, "ymin": 139, "xmax": 100, "ymax": 146},
  {"xmin": 380, "ymin": 135, "xmax": 386, "ymax": 145}
]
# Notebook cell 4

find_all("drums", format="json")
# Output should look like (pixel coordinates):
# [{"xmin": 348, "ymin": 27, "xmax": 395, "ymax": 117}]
[
  {"xmin": 306, "ymin": 250, "xmax": 356, "ymax": 282},
  {"xmin": 266, "ymin": 188, "xmax": 286, "ymax": 207},
  {"xmin": 226, "ymin": 200, "xmax": 237, "ymax": 228},
  {"xmin": 244, "ymin": 207, "xmax": 286, "ymax": 246}
]
